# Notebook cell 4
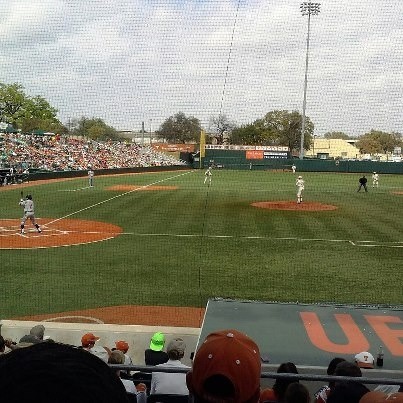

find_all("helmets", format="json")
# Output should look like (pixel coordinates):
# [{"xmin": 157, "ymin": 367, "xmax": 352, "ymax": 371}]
[{"xmin": 26, "ymin": 195, "xmax": 31, "ymax": 199}]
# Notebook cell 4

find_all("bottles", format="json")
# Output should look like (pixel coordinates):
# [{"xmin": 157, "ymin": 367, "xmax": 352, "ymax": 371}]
[{"xmin": 377, "ymin": 346, "xmax": 384, "ymax": 366}]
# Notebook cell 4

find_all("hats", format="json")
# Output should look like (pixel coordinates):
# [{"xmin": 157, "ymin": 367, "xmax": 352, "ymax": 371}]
[
  {"xmin": 355, "ymin": 352, "xmax": 374, "ymax": 364},
  {"xmin": 81, "ymin": 333, "xmax": 99, "ymax": 345},
  {"xmin": 192, "ymin": 329, "xmax": 261, "ymax": 403},
  {"xmin": 115, "ymin": 341, "xmax": 129, "ymax": 350},
  {"xmin": 111, "ymin": 351, "xmax": 125, "ymax": 363},
  {"xmin": 167, "ymin": 337, "xmax": 185, "ymax": 355},
  {"xmin": 150, "ymin": 332, "xmax": 165, "ymax": 351},
  {"xmin": 30, "ymin": 325, "xmax": 45, "ymax": 338}
]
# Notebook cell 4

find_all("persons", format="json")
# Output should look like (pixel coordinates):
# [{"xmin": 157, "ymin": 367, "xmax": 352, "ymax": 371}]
[
  {"xmin": 292, "ymin": 164, "xmax": 296, "ymax": 175},
  {"xmin": 88, "ymin": 168, "xmax": 94, "ymax": 187},
  {"xmin": 335, "ymin": 159, "xmax": 340, "ymax": 172},
  {"xmin": 371, "ymin": 172, "xmax": 379, "ymax": 188},
  {"xmin": 296, "ymin": 175, "xmax": 305, "ymax": 204},
  {"xmin": 357, "ymin": 175, "xmax": 368, "ymax": 193},
  {"xmin": 0, "ymin": 325, "xmax": 403, "ymax": 403},
  {"xmin": 203, "ymin": 167, "xmax": 212, "ymax": 185},
  {"xmin": 0, "ymin": 130, "xmax": 188, "ymax": 186},
  {"xmin": 19, "ymin": 194, "xmax": 42, "ymax": 235}
]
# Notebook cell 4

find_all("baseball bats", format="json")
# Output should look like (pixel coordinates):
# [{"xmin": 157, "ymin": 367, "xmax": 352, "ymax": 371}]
[{"xmin": 19, "ymin": 190, "xmax": 24, "ymax": 202}]
